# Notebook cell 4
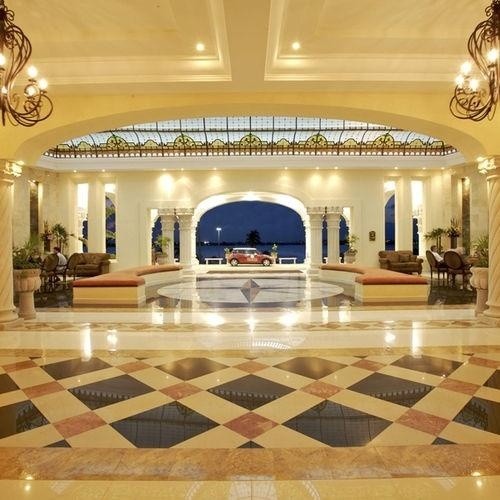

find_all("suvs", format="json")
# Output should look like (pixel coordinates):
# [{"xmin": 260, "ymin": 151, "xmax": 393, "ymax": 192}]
[{"xmin": 227, "ymin": 247, "xmax": 274, "ymax": 267}]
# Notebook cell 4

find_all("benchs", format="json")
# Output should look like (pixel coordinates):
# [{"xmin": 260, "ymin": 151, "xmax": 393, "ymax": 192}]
[
  {"xmin": 72, "ymin": 264, "xmax": 196, "ymax": 309},
  {"xmin": 278, "ymin": 258, "xmax": 298, "ymax": 264},
  {"xmin": 205, "ymin": 258, "xmax": 223, "ymax": 265},
  {"xmin": 319, "ymin": 264, "xmax": 431, "ymax": 303}
]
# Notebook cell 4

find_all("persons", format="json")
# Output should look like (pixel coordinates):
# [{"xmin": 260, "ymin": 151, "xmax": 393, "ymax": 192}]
[
  {"xmin": 53, "ymin": 247, "xmax": 68, "ymax": 283},
  {"xmin": 430, "ymin": 245, "xmax": 447, "ymax": 269},
  {"xmin": 455, "ymin": 247, "xmax": 475, "ymax": 292}
]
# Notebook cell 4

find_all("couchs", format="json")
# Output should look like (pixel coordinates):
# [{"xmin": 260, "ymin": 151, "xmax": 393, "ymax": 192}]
[
  {"xmin": 65, "ymin": 253, "xmax": 111, "ymax": 281},
  {"xmin": 378, "ymin": 250, "xmax": 423, "ymax": 276}
]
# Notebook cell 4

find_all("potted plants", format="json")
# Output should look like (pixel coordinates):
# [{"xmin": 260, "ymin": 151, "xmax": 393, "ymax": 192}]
[
  {"xmin": 446, "ymin": 227, "xmax": 462, "ymax": 249},
  {"xmin": 12, "ymin": 238, "xmax": 46, "ymax": 320},
  {"xmin": 467, "ymin": 231, "xmax": 491, "ymax": 290},
  {"xmin": 343, "ymin": 234, "xmax": 360, "ymax": 263}
]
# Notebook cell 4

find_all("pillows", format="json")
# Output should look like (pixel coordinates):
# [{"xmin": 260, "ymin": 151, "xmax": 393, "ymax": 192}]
[{"xmin": 399, "ymin": 253, "xmax": 410, "ymax": 263}]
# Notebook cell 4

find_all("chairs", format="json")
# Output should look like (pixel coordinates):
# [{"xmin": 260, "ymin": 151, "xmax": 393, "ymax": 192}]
[
  {"xmin": 40, "ymin": 252, "xmax": 81, "ymax": 292},
  {"xmin": 426, "ymin": 250, "xmax": 473, "ymax": 294}
]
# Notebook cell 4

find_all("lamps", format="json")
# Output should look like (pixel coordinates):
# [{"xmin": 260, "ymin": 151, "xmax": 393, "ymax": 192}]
[
  {"xmin": 0, "ymin": 1, "xmax": 55, "ymax": 130},
  {"xmin": 447, "ymin": 1, "xmax": 500, "ymax": 122}
]
